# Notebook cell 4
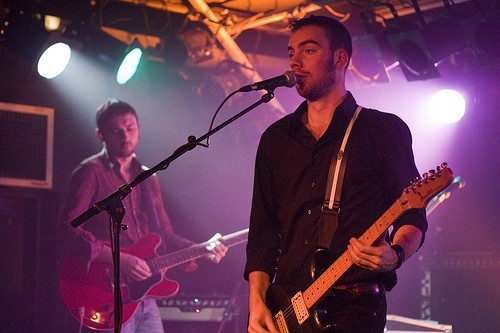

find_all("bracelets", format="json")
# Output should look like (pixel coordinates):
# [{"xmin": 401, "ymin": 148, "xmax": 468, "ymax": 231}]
[{"xmin": 391, "ymin": 245, "xmax": 404, "ymax": 270}]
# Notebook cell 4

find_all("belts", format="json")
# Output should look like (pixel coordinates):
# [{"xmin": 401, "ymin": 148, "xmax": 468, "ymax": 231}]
[{"xmin": 329, "ymin": 283, "xmax": 386, "ymax": 298}]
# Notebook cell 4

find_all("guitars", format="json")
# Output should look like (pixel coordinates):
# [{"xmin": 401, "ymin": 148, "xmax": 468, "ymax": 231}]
[
  {"xmin": 53, "ymin": 227, "xmax": 250, "ymax": 331},
  {"xmin": 262, "ymin": 162, "xmax": 454, "ymax": 333}
]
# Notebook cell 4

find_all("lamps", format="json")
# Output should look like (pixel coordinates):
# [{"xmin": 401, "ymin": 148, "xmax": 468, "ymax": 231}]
[
  {"xmin": 397, "ymin": 33, "xmax": 449, "ymax": 83},
  {"xmin": 348, "ymin": 32, "xmax": 390, "ymax": 89},
  {"xmin": 160, "ymin": 26, "xmax": 214, "ymax": 69},
  {"xmin": 11, "ymin": 27, "xmax": 73, "ymax": 79},
  {"xmin": 83, "ymin": 33, "xmax": 143, "ymax": 86}
]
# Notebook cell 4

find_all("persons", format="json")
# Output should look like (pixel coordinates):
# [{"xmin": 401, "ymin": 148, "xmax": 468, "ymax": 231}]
[
  {"xmin": 57, "ymin": 98, "xmax": 228, "ymax": 333},
  {"xmin": 244, "ymin": 15, "xmax": 429, "ymax": 333}
]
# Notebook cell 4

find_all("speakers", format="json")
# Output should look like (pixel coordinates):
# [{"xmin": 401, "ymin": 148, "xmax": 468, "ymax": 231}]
[{"xmin": 0, "ymin": 98, "xmax": 58, "ymax": 199}]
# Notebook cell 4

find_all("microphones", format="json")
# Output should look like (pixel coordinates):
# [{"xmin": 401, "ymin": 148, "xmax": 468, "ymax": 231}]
[{"xmin": 240, "ymin": 71, "xmax": 299, "ymax": 92}]
